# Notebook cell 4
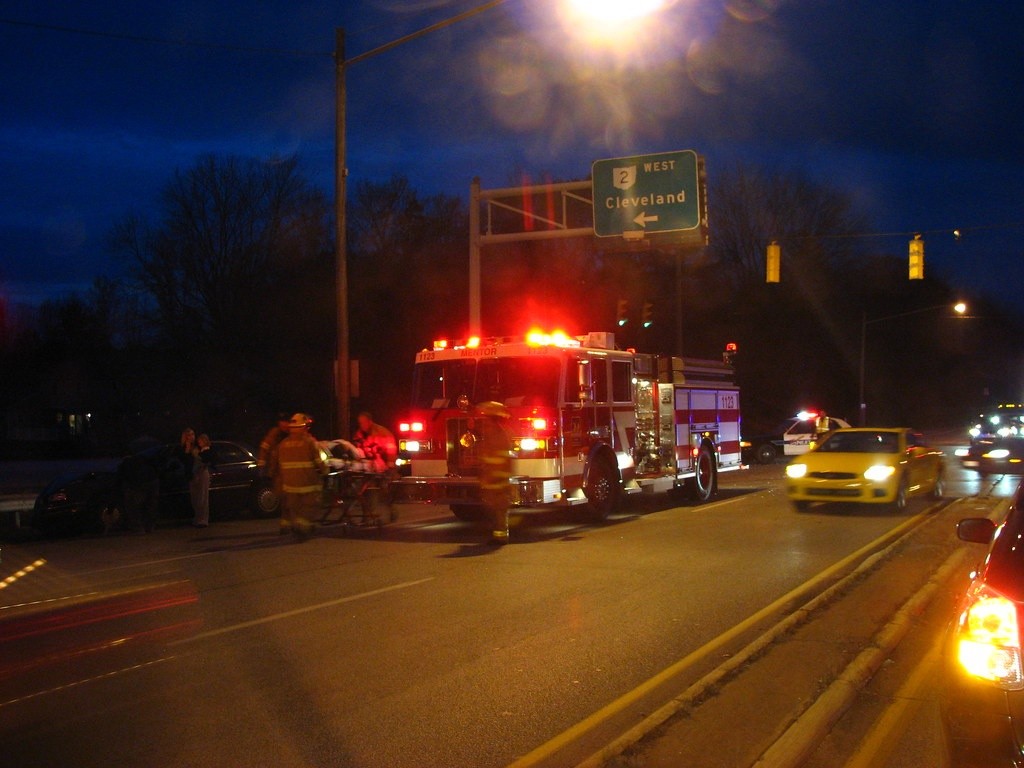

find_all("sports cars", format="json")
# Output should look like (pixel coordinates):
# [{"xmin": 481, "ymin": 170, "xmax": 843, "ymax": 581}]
[{"xmin": 782, "ymin": 426, "xmax": 951, "ymax": 512}]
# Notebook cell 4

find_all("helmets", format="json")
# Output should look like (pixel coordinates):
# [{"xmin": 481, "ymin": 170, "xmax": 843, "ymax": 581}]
[
  {"xmin": 288, "ymin": 414, "xmax": 309, "ymax": 427},
  {"xmin": 477, "ymin": 401, "xmax": 508, "ymax": 418}
]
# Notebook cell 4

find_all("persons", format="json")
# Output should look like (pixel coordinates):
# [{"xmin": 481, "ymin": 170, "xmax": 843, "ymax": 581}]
[
  {"xmin": 175, "ymin": 410, "xmax": 403, "ymax": 541},
  {"xmin": 810, "ymin": 409, "xmax": 832, "ymax": 451},
  {"xmin": 479, "ymin": 401, "xmax": 516, "ymax": 542}
]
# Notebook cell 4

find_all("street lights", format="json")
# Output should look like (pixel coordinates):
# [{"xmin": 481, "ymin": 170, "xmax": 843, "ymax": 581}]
[
  {"xmin": 859, "ymin": 295, "xmax": 971, "ymax": 432},
  {"xmin": 335, "ymin": 0, "xmax": 670, "ymax": 457}
]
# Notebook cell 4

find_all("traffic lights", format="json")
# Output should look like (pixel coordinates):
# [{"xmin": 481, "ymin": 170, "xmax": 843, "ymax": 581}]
[
  {"xmin": 614, "ymin": 297, "xmax": 630, "ymax": 331},
  {"xmin": 638, "ymin": 301, "xmax": 654, "ymax": 330}
]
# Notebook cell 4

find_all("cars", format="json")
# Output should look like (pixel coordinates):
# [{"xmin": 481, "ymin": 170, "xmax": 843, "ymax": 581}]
[
  {"xmin": 939, "ymin": 473, "xmax": 1024, "ymax": 768},
  {"xmin": 741, "ymin": 411, "xmax": 884, "ymax": 467},
  {"xmin": 972, "ymin": 400, "xmax": 1024, "ymax": 475},
  {"xmin": 43, "ymin": 438, "xmax": 284, "ymax": 534}
]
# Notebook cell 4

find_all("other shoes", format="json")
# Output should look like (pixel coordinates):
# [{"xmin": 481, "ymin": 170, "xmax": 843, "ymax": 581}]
[
  {"xmin": 280, "ymin": 528, "xmax": 287, "ymax": 534},
  {"xmin": 293, "ymin": 528, "xmax": 307, "ymax": 538}
]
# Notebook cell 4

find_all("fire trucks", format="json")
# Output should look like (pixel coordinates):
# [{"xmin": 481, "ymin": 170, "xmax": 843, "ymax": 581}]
[{"xmin": 391, "ymin": 325, "xmax": 751, "ymax": 525}]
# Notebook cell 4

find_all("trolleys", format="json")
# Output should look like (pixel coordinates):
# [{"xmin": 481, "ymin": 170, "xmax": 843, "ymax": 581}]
[{"xmin": 312, "ymin": 464, "xmax": 402, "ymax": 529}]
